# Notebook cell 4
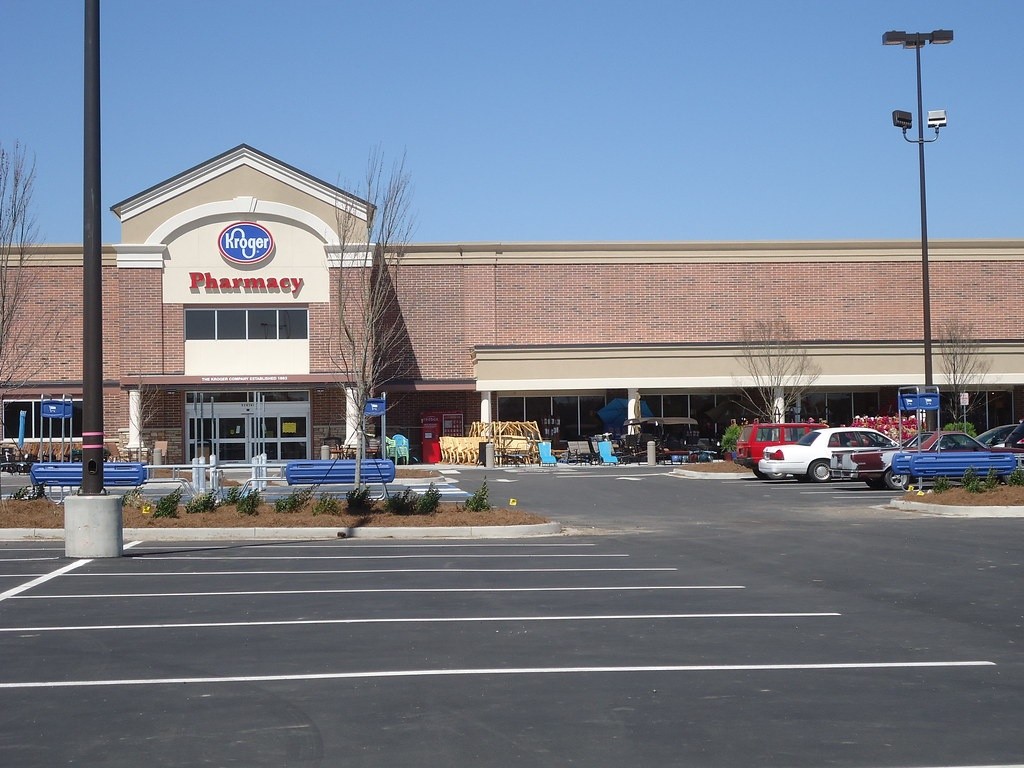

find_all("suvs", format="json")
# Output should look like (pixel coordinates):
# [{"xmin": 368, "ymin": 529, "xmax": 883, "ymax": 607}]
[{"xmin": 732, "ymin": 417, "xmax": 830, "ymax": 480}]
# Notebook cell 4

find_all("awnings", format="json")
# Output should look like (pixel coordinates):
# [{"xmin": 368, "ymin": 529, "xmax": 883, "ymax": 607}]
[{"xmin": 624, "ymin": 417, "xmax": 700, "ymax": 464}]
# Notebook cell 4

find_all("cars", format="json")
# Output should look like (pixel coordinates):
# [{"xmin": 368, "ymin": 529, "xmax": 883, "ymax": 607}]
[
  {"xmin": 974, "ymin": 418, "xmax": 1024, "ymax": 448},
  {"xmin": 758, "ymin": 427, "xmax": 905, "ymax": 483}
]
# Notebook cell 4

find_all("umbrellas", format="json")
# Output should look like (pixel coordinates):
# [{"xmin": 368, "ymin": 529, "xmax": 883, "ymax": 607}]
[{"xmin": 598, "ymin": 398, "xmax": 654, "ymax": 435}]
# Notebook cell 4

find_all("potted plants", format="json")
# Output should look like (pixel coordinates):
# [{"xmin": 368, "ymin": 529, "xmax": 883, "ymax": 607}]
[{"xmin": 720, "ymin": 423, "xmax": 742, "ymax": 461}]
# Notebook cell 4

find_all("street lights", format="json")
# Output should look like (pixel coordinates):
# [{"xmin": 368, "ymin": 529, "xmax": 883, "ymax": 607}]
[{"xmin": 882, "ymin": 28, "xmax": 954, "ymax": 431}]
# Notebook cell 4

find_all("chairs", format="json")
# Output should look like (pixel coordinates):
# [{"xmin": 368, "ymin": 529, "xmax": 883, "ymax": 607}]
[
  {"xmin": 567, "ymin": 441, "xmax": 619, "ymax": 466},
  {"xmin": 538, "ymin": 442, "xmax": 557, "ymax": 467},
  {"xmin": 393, "ymin": 434, "xmax": 409, "ymax": 465},
  {"xmin": 366, "ymin": 437, "xmax": 381, "ymax": 459},
  {"xmin": 439, "ymin": 436, "xmax": 531, "ymax": 466},
  {"xmin": 151, "ymin": 441, "xmax": 168, "ymax": 465},
  {"xmin": 386, "ymin": 436, "xmax": 397, "ymax": 466},
  {"xmin": 323, "ymin": 437, "xmax": 342, "ymax": 460},
  {"xmin": 107, "ymin": 443, "xmax": 129, "ymax": 462}
]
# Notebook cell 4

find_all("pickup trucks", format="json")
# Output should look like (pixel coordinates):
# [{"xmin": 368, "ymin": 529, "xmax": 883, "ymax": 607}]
[{"xmin": 828, "ymin": 431, "xmax": 1024, "ymax": 490}]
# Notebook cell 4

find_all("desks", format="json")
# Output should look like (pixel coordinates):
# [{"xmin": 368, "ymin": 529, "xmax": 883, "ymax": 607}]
[{"xmin": 342, "ymin": 447, "xmax": 356, "ymax": 459}]
